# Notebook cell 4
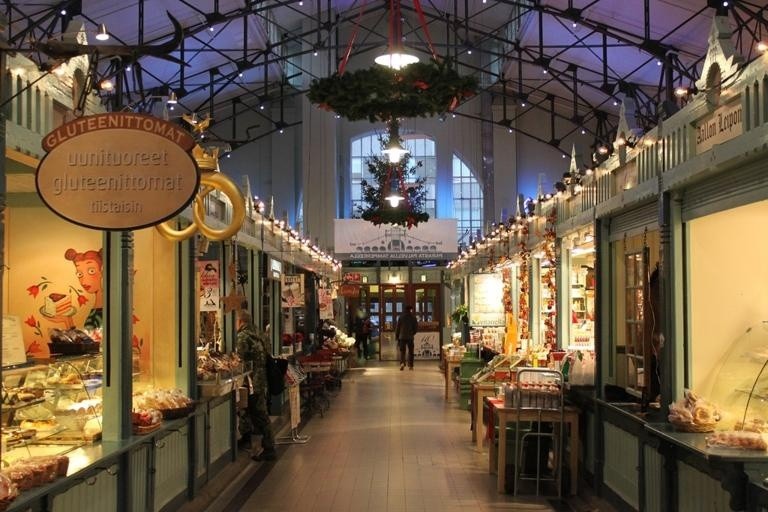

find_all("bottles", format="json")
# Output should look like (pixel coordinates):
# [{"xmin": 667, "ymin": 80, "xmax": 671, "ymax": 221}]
[
  {"xmin": 504, "ymin": 380, "xmax": 561, "ymax": 409},
  {"xmin": 532, "ymin": 350, "xmax": 538, "ymax": 368}
]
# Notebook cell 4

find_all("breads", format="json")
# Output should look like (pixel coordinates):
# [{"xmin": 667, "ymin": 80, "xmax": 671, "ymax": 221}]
[
  {"xmin": 667, "ymin": 388, "xmax": 768, "ymax": 450},
  {"xmin": 0, "ymin": 369, "xmax": 192, "ymax": 512}
]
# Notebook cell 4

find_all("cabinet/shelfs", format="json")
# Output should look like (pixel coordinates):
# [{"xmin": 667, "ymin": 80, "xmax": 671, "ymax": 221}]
[
  {"xmin": 708, "ymin": 322, "xmax": 767, "ymax": 438},
  {"xmin": 2, "ymin": 347, "xmax": 150, "ymax": 473}
]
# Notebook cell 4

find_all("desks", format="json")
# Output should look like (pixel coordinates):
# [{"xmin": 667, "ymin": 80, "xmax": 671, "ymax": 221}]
[{"xmin": 444, "ymin": 348, "xmax": 582, "ymax": 496}]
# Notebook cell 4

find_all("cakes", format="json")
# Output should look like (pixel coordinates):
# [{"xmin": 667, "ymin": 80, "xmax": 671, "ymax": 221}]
[{"xmin": 45, "ymin": 293, "xmax": 71, "ymax": 316}]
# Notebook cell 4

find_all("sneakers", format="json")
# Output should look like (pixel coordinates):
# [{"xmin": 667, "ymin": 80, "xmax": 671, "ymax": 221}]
[
  {"xmin": 400, "ymin": 363, "xmax": 413, "ymax": 371},
  {"xmin": 238, "ymin": 436, "xmax": 277, "ymax": 461}
]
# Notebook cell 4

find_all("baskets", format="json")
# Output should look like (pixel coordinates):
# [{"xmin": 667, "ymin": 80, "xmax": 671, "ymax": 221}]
[
  {"xmin": 47, "ymin": 340, "xmax": 101, "ymax": 355},
  {"xmin": 158, "ymin": 401, "xmax": 197, "ymax": 421},
  {"xmin": 676, "ymin": 407, "xmax": 717, "ymax": 433}
]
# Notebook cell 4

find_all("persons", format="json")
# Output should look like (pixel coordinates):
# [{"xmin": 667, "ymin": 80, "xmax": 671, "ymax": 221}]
[
  {"xmin": 393, "ymin": 304, "xmax": 417, "ymax": 372},
  {"xmin": 234, "ymin": 307, "xmax": 278, "ymax": 462},
  {"xmin": 63, "ymin": 246, "xmax": 104, "ymax": 345},
  {"xmin": 354, "ymin": 314, "xmax": 372, "ymax": 359}
]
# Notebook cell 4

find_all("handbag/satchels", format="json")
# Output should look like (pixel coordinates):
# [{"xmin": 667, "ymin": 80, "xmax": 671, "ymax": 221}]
[{"xmin": 268, "ymin": 356, "xmax": 289, "ymax": 396}]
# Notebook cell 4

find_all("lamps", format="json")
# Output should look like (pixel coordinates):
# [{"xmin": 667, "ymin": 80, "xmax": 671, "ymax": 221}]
[
  {"xmin": 41, "ymin": 23, "xmax": 110, "ymax": 41},
  {"xmin": 375, "ymin": 1, "xmax": 420, "ymax": 70},
  {"xmin": 118, "ymin": 90, "xmax": 178, "ymax": 113}
]
